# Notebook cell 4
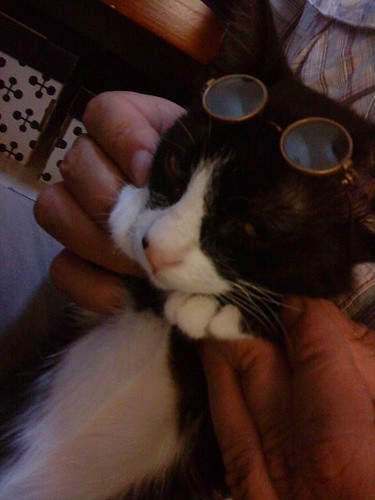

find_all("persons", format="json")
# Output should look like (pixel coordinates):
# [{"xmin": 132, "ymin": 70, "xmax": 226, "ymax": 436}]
[{"xmin": 32, "ymin": 90, "xmax": 375, "ymax": 500}]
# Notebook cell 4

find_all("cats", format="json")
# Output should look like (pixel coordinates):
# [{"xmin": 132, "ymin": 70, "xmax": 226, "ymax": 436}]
[{"xmin": 0, "ymin": 0, "xmax": 375, "ymax": 500}]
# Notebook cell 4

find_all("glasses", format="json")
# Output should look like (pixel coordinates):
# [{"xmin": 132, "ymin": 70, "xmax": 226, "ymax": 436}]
[{"xmin": 201, "ymin": 72, "xmax": 369, "ymax": 195}]
